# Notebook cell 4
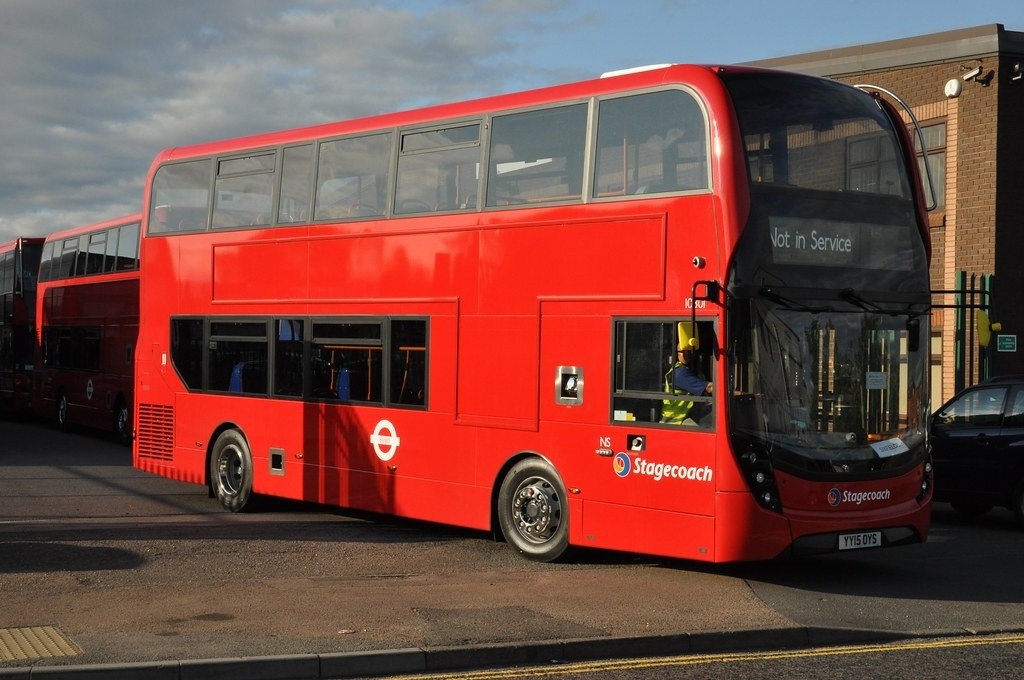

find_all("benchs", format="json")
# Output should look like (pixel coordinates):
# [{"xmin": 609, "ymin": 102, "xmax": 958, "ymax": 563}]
[{"xmin": 176, "ymin": 195, "xmax": 528, "ymax": 231}]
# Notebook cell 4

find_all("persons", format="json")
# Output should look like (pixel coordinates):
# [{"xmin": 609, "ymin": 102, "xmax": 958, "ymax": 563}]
[{"xmin": 659, "ymin": 344, "xmax": 764, "ymax": 425}]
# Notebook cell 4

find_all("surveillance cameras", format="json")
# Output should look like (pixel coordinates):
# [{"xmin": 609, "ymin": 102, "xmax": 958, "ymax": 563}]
[{"xmin": 961, "ymin": 66, "xmax": 984, "ymax": 81}]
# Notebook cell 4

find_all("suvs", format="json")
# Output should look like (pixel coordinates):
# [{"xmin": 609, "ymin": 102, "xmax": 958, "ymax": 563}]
[{"xmin": 927, "ymin": 376, "xmax": 1024, "ymax": 530}]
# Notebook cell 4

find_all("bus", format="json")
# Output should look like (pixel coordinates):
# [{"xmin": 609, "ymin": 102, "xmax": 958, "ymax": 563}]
[
  {"xmin": 36, "ymin": 208, "xmax": 254, "ymax": 446},
  {"xmin": 0, "ymin": 237, "xmax": 46, "ymax": 419},
  {"xmin": 131, "ymin": 64, "xmax": 1002, "ymax": 563}
]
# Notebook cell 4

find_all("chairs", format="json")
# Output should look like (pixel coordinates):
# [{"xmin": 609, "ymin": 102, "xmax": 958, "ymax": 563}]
[
  {"xmin": 339, "ymin": 370, "xmax": 363, "ymax": 402},
  {"xmin": 229, "ymin": 362, "xmax": 267, "ymax": 392}
]
